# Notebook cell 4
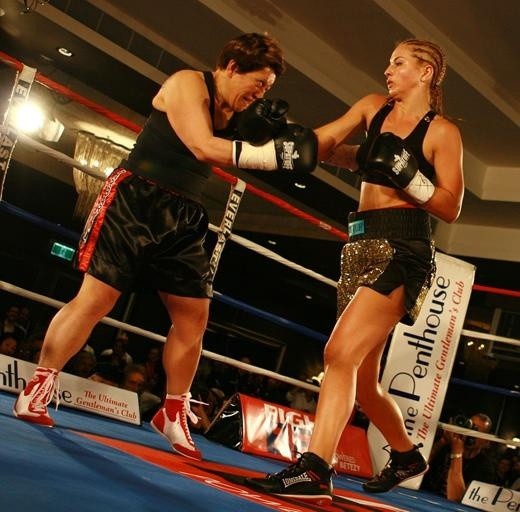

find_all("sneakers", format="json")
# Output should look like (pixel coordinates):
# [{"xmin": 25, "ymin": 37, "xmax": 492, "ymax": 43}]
[
  {"xmin": 245, "ymin": 452, "xmax": 337, "ymax": 507},
  {"xmin": 361, "ymin": 444, "xmax": 430, "ymax": 494}
]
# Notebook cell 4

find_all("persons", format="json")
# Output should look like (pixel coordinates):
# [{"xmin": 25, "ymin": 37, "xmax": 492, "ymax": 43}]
[
  {"xmin": 248, "ymin": 37, "xmax": 467, "ymax": 504},
  {"xmin": 65, "ymin": 334, "xmax": 165, "ymax": 417},
  {"xmin": 14, "ymin": 32, "xmax": 320, "ymax": 460},
  {"xmin": 185, "ymin": 359, "xmax": 290, "ymax": 437},
  {"xmin": 422, "ymin": 415, "xmax": 518, "ymax": 501},
  {"xmin": 1, "ymin": 300, "xmax": 44, "ymax": 362}
]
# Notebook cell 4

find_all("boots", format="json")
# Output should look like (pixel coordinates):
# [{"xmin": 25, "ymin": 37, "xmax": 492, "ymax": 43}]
[
  {"xmin": 149, "ymin": 393, "xmax": 203, "ymax": 461},
  {"xmin": 12, "ymin": 366, "xmax": 61, "ymax": 428}
]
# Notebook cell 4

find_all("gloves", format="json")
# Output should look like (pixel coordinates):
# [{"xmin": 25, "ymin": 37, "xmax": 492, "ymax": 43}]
[
  {"xmin": 366, "ymin": 130, "xmax": 435, "ymax": 205},
  {"xmin": 227, "ymin": 123, "xmax": 317, "ymax": 173},
  {"xmin": 236, "ymin": 98, "xmax": 289, "ymax": 148}
]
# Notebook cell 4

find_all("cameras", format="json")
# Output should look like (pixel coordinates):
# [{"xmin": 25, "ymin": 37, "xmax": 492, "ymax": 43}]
[{"xmin": 453, "ymin": 415, "xmax": 478, "ymax": 444}]
